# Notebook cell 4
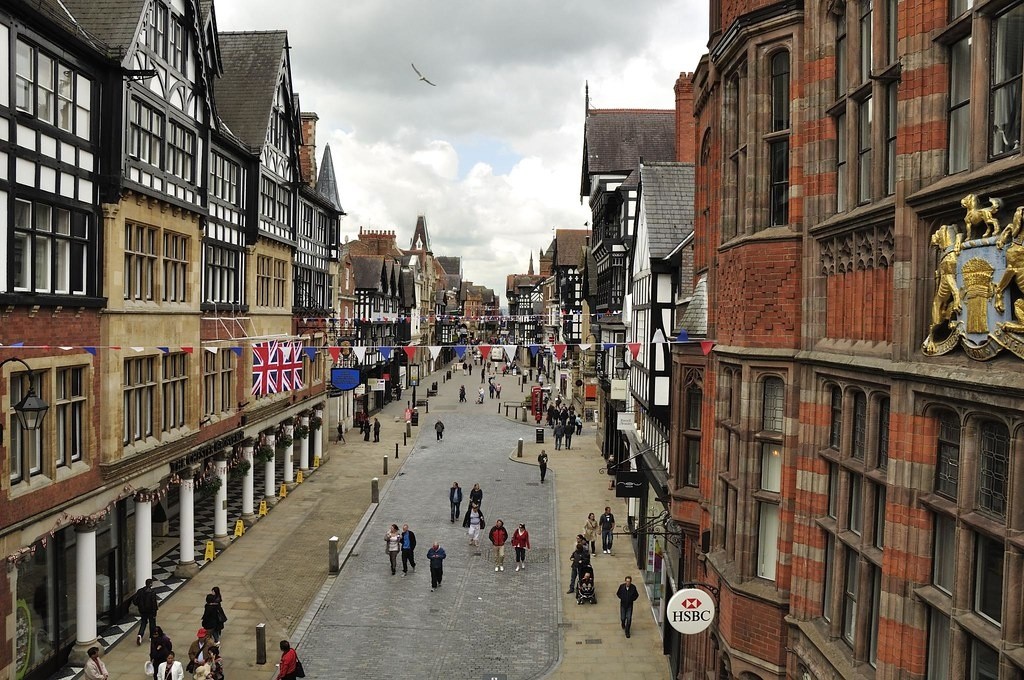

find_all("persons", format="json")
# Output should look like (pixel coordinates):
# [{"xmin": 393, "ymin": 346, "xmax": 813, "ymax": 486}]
[
  {"xmin": 426, "ymin": 542, "xmax": 446, "ymax": 591},
  {"xmin": 202, "ymin": 587, "xmax": 227, "ymax": 642},
  {"xmin": 538, "ymin": 450, "xmax": 548, "ymax": 481},
  {"xmin": 568, "ymin": 534, "xmax": 591, "ymax": 594},
  {"xmin": 335, "ymin": 410, "xmax": 381, "ymax": 444},
  {"xmin": 599, "ymin": 507, "xmax": 615, "ymax": 553},
  {"xmin": 458, "ymin": 338, "xmax": 551, "ymax": 404},
  {"xmin": 84, "ymin": 647, "xmax": 108, "ymax": 680},
  {"xmin": 134, "ymin": 579, "xmax": 159, "ymax": 646},
  {"xmin": 535, "ymin": 394, "xmax": 583, "ymax": 450},
  {"xmin": 150, "ymin": 626, "xmax": 224, "ymax": 680},
  {"xmin": 434, "ymin": 420, "xmax": 444, "ymax": 442},
  {"xmin": 450, "ymin": 481, "xmax": 485, "ymax": 545},
  {"xmin": 617, "ymin": 576, "xmax": 639, "ymax": 638},
  {"xmin": 275, "ymin": 640, "xmax": 297, "ymax": 680},
  {"xmin": 488, "ymin": 519, "xmax": 508, "ymax": 571},
  {"xmin": 384, "ymin": 524, "xmax": 416, "ymax": 577},
  {"xmin": 396, "ymin": 385, "xmax": 401, "ymax": 400},
  {"xmin": 583, "ymin": 513, "xmax": 599, "ymax": 556},
  {"xmin": 511, "ymin": 523, "xmax": 530, "ymax": 571}
]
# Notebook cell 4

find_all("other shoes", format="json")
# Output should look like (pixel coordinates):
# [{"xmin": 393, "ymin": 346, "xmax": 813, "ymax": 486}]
[
  {"xmin": 149, "ymin": 637, "xmax": 151, "ymax": 642},
  {"xmin": 431, "ymin": 587, "xmax": 437, "ymax": 592},
  {"xmin": 522, "ymin": 563, "xmax": 525, "ymax": 569},
  {"xmin": 625, "ymin": 633, "xmax": 630, "ymax": 638},
  {"xmin": 392, "ymin": 572, "xmax": 396, "ymax": 575},
  {"xmin": 450, "ymin": 518, "xmax": 611, "ymax": 556},
  {"xmin": 494, "ymin": 567, "xmax": 499, "ymax": 572},
  {"xmin": 500, "ymin": 566, "xmax": 504, "ymax": 571},
  {"xmin": 567, "ymin": 590, "xmax": 573, "ymax": 594},
  {"xmin": 622, "ymin": 623, "xmax": 625, "ymax": 629},
  {"xmin": 515, "ymin": 565, "xmax": 520, "ymax": 572},
  {"xmin": 214, "ymin": 641, "xmax": 221, "ymax": 646},
  {"xmin": 401, "ymin": 572, "xmax": 407, "ymax": 578},
  {"xmin": 136, "ymin": 635, "xmax": 142, "ymax": 646},
  {"xmin": 438, "ymin": 582, "xmax": 442, "ymax": 586},
  {"xmin": 413, "ymin": 564, "xmax": 417, "ymax": 573}
]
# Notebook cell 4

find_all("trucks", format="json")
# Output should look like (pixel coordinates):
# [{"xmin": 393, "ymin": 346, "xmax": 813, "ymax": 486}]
[{"xmin": 491, "ymin": 348, "xmax": 504, "ymax": 362}]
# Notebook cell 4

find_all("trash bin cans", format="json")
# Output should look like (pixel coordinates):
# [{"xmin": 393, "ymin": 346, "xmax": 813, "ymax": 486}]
[
  {"xmin": 447, "ymin": 371, "xmax": 451, "ymax": 379},
  {"xmin": 411, "ymin": 412, "xmax": 418, "ymax": 426},
  {"xmin": 522, "ymin": 375, "xmax": 527, "ymax": 384},
  {"xmin": 536, "ymin": 428, "xmax": 544, "ymax": 443}
]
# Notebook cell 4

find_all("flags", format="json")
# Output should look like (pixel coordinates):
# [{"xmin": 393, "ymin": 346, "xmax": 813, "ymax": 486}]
[{"xmin": 251, "ymin": 340, "xmax": 304, "ymax": 395}]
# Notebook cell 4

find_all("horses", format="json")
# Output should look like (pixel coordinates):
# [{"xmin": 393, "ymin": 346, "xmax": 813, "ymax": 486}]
[
  {"xmin": 928, "ymin": 206, "xmax": 1024, "ymax": 358},
  {"xmin": 961, "ymin": 194, "xmax": 1001, "ymax": 241}
]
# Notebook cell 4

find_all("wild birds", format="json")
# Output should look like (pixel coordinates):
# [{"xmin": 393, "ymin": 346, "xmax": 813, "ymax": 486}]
[{"xmin": 412, "ymin": 64, "xmax": 436, "ymax": 86}]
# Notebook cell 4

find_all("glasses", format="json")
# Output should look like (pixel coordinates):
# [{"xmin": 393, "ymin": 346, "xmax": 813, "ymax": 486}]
[
  {"xmin": 152, "ymin": 633, "xmax": 158, "ymax": 635},
  {"xmin": 519, "ymin": 526, "xmax": 523, "ymax": 528},
  {"xmin": 208, "ymin": 652, "xmax": 211, "ymax": 655}
]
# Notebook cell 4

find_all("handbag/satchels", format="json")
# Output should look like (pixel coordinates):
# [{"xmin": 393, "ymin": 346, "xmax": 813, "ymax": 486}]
[
  {"xmin": 186, "ymin": 660, "xmax": 194, "ymax": 674},
  {"xmin": 296, "ymin": 656, "xmax": 305, "ymax": 678},
  {"xmin": 217, "ymin": 606, "xmax": 227, "ymax": 623},
  {"xmin": 144, "ymin": 661, "xmax": 154, "ymax": 676}
]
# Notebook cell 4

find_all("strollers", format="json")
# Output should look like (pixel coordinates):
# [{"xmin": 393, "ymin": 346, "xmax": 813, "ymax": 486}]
[{"xmin": 576, "ymin": 565, "xmax": 597, "ymax": 605}]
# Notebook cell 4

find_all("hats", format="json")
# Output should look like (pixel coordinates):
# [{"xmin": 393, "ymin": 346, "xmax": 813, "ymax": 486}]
[
  {"xmin": 197, "ymin": 628, "xmax": 207, "ymax": 638},
  {"xmin": 193, "ymin": 663, "xmax": 211, "ymax": 680}
]
